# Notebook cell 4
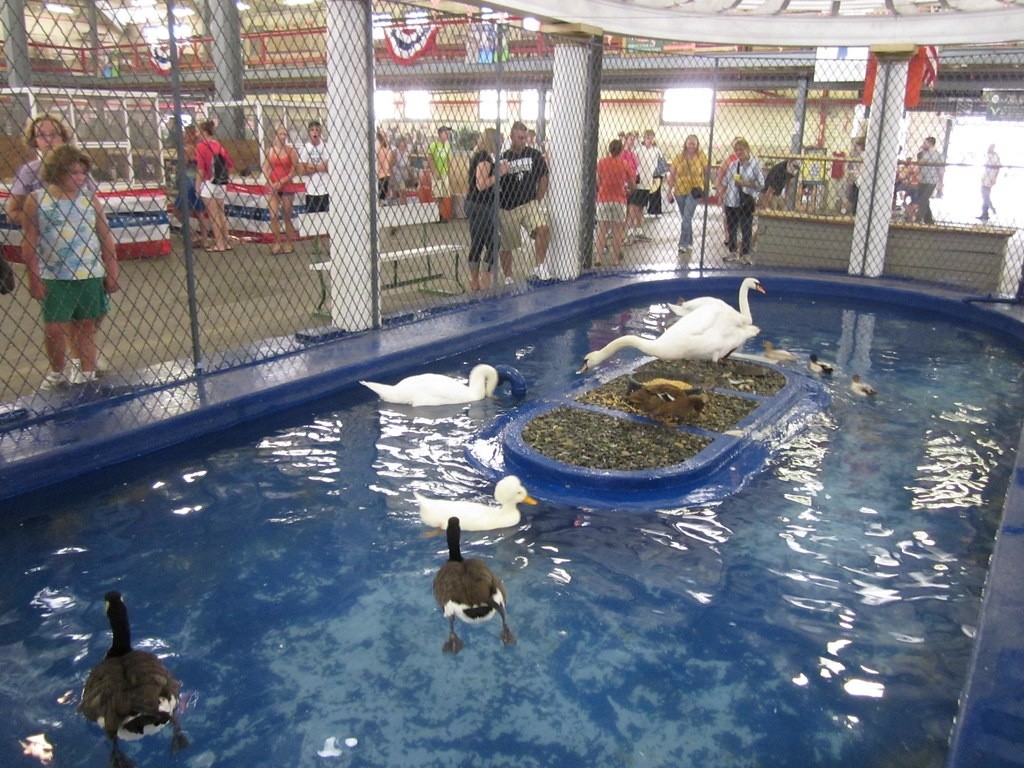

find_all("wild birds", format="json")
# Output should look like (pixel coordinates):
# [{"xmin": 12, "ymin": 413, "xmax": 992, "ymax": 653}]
[
  {"xmin": 432, "ymin": 517, "xmax": 517, "ymax": 652},
  {"xmin": 762, "ymin": 341, "xmax": 794, "ymax": 360},
  {"xmin": 75, "ymin": 590, "xmax": 192, "ymax": 768},
  {"xmin": 850, "ymin": 374, "xmax": 877, "ymax": 397},
  {"xmin": 809, "ymin": 354, "xmax": 834, "ymax": 373}
]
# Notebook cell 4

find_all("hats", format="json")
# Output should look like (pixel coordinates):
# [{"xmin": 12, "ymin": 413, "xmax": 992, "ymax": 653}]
[{"xmin": 438, "ymin": 126, "xmax": 452, "ymax": 134}]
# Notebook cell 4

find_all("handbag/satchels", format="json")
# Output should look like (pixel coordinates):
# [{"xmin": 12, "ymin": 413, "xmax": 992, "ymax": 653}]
[
  {"xmin": 738, "ymin": 191, "xmax": 755, "ymax": 213},
  {"xmin": 693, "ymin": 187, "xmax": 703, "ymax": 199}
]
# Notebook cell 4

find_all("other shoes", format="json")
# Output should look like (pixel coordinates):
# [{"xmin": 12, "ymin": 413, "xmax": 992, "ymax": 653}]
[
  {"xmin": 30, "ymin": 376, "xmax": 67, "ymax": 393},
  {"xmin": 644, "ymin": 214, "xmax": 662, "ymax": 218},
  {"xmin": 678, "ymin": 247, "xmax": 687, "ymax": 253},
  {"xmin": 533, "ymin": 263, "xmax": 552, "ymax": 281},
  {"xmin": 69, "ymin": 359, "xmax": 83, "ymax": 384},
  {"xmin": 739, "ymin": 253, "xmax": 752, "ymax": 265},
  {"xmin": 506, "ymin": 276, "xmax": 514, "ymax": 285},
  {"xmin": 723, "ymin": 252, "xmax": 739, "ymax": 261}
]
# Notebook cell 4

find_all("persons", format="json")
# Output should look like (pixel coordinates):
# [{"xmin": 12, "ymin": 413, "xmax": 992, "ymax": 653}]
[
  {"xmin": 465, "ymin": 121, "xmax": 553, "ymax": 290},
  {"xmin": 716, "ymin": 135, "xmax": 764, "ymax": 266},
  {"xmin": 758, "ymin": 159, "xmax": 802, "ymax": 210},
  {"xmin": 7, "ymin": 115, "xmax": 119, "ymax": 389},
  {"xmin": 596, "ymin": 129, "xmax": 666, "ymax": 266},
  {"xmin": 175, "ymin": 122, "xmax": 235, "ymax": 252},
  {"xmin": 668, "ymin": 135, "xmax": 708, "ymax": 252},
  {"xmin": 977, "ymin": 143, "xmax": 1001, "ymax": 219},
  {"xmin": 377, "ymin": 132, "xmax": 408, "ymax": 200},
  {"xmin": 428, "ymin": 126, "xmax": 453, "ymax": 224},
  {"xmin": 893, "ymin": 137, "xmax": 945, "ymax": 221},
  {"xmin": 263, "ymin": 120, "xmax": 329, "ymax": 257}
]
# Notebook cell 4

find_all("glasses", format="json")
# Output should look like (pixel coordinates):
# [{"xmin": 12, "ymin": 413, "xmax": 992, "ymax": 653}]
[{"xmin": 34, "ymin": 131, "xmax": 59, "ymax": 140}]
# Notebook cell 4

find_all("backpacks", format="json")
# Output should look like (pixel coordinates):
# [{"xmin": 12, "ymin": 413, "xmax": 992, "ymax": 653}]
[{"xmin": 201, "ymin": 141, "xmax": 229, "ymax": 185}]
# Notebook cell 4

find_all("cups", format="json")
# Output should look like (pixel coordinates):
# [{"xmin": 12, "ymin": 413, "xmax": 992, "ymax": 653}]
[{"xmin": 733, "ymin": 173, "xmax": 740, "ymax": 181}]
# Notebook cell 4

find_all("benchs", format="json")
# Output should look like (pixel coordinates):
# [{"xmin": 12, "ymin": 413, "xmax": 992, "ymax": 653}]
[{"xmin": 298, "ymin": 202, "xmax": 467, "ymax": 317}]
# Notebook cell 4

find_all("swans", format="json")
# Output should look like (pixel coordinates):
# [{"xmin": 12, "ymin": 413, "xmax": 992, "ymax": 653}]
[
  {"xmin": 670, "ymin": 278, "xmax": 765, "ymax": 324},
  {"xmin": 412, "ymin": 475, "xmax": 539, "ymax": 532},
  {"xmin": 358, "ymin": 363, "xmax": 499, "ymax": 407},
  {"xmin": 574, "ymin": 304, "xmax": 762, "ymax": 376}
]
